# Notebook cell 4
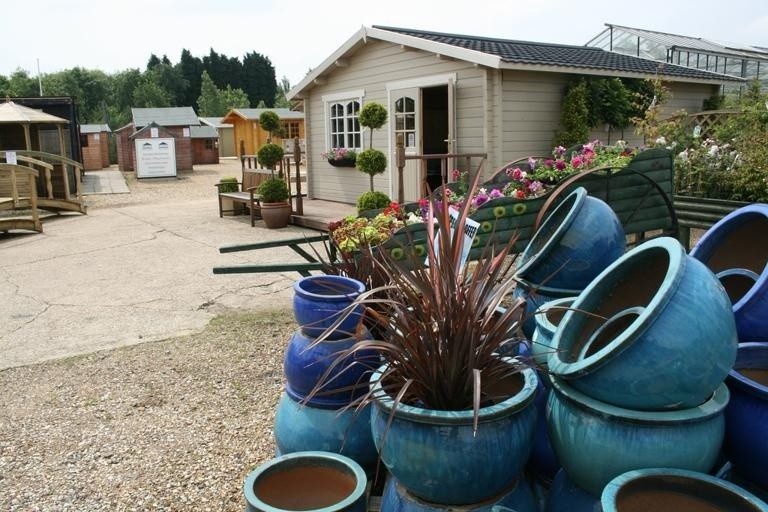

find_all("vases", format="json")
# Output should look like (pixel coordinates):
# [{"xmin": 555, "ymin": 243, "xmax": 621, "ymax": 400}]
[{"xmin": 242, "ymin": 157, "xmax": 768, "ymax": 512}]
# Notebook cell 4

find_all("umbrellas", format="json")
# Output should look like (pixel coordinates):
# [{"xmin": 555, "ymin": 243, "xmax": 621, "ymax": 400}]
[{"xmin": 0, "ymin": 101, "xmax": 71, "ymax": 125}]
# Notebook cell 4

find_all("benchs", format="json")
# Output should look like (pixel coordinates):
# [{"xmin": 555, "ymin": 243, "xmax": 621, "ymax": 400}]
[{"xmin": 213, "ymin": 169, "xmax": 281, "ymax": 227}]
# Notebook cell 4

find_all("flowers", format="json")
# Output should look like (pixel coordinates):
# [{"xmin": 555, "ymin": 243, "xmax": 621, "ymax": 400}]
[{"xmin": 328, "ymin": 141, "xmax": 649, "ymax": 252}]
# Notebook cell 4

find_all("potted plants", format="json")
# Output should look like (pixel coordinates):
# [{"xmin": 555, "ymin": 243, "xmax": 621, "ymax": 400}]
[
  {"xmin": 219, "ymin": 176, "xmax": 242, "ymax": 216},
  {"xmin": 256, "ymin": 111, "xmax": 291, "ymax": 229}
]
